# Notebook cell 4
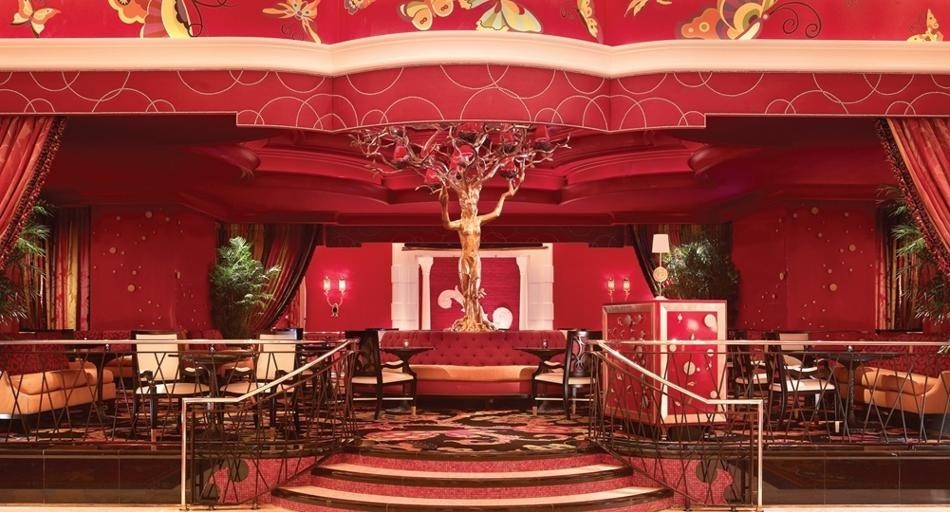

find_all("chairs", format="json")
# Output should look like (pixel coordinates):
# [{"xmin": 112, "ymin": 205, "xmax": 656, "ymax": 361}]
[
  {"xmin": 0, "ymin": 327, "xmax": 604, "ymax": 443},
  {"xmin": 728, "ymin": 327, "xmax": 950, "ymax": 438}
]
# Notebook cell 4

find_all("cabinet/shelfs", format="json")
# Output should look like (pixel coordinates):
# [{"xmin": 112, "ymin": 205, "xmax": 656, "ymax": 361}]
[{"xmin": 600, "ymin": 299, "xmax": 728, "ymax": 439}]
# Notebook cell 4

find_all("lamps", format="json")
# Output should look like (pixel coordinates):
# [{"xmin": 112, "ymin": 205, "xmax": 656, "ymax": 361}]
[
  {"xmin": 608, "ymin": 274, "xmax": 631, "ymax": 304},
  {"xmin": 322, "ymin": 276, "xmax": 347, "ymax": 319},
  {"xmin": 651, "ymin": 233, "xmax": 671, "ymax": 301}
]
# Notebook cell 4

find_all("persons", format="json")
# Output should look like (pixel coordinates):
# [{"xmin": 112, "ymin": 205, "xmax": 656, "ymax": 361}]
[{"xmin": 439, "ymin": 180, "xmax": 518, "ymax": 315}]
